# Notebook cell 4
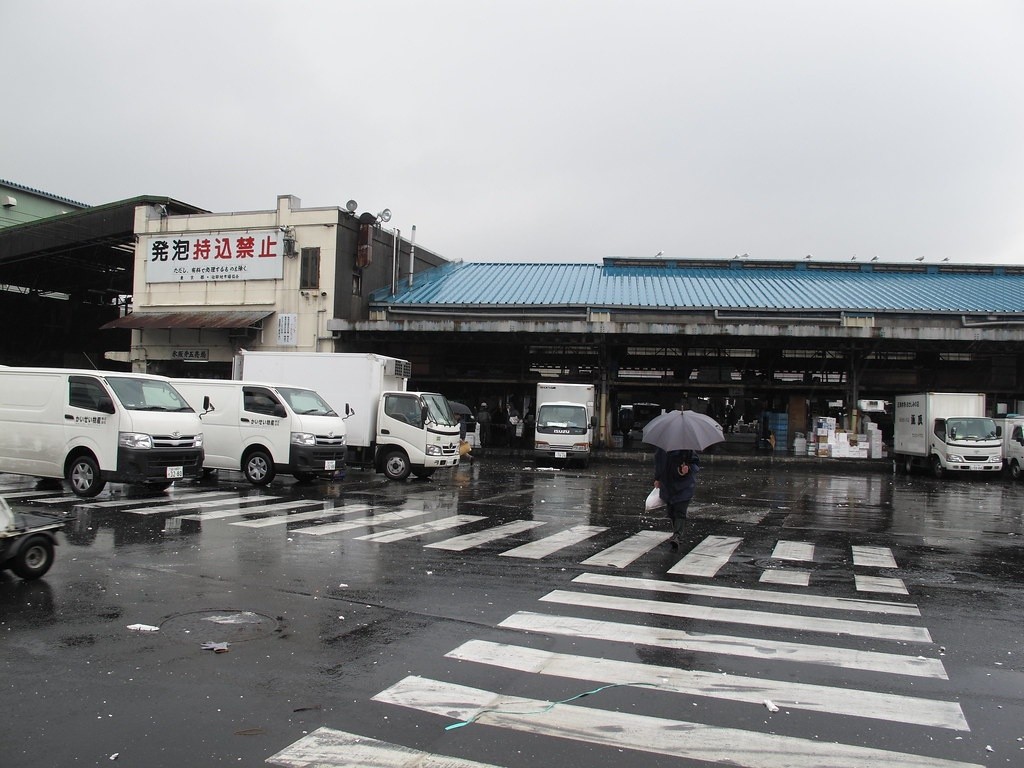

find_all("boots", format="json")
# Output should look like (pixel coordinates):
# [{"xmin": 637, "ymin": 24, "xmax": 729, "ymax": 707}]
[
  {"xmin": 678, "ymin": 519, "xmax": 685, "ymax": 543},
  {"xmin": 670, "ymin": 520, "xmax": 679, "ymax": 548}
]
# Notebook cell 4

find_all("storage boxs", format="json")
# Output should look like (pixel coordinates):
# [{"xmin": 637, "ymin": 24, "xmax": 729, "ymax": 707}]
[
  {"xmin": 792, "ymin": 416, "xmax": 887, "ymax": 458},
  {"xmin": 613, "ymin": 436, "xmax": 623, "ymax": 448},
  {"xmin": 768, "ymin": 413, "xmax": 788, "ymax": 450}
]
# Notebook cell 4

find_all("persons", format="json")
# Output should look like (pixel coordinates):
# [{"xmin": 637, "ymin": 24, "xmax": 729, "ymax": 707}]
[
  {"xmin": 654, "ymin": 447, "xmax": 700, "ymax": 548},
  {"xmin": 477, "ymin": 407, "xmax": 492, "ymax": 445},
  {"xmin": 505, "ymin": 403, "xmax": 519, "ymax": 446},
  {"xmin": 524, "ymin": 398, "xmax": 536, "ymax": 421},
  {"xmin": 725, "ymin": 405, "xmax": 738, "ymax": 436}
]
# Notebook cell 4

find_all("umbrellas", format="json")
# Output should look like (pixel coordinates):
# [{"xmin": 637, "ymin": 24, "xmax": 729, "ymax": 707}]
[{"xmin": 642, "ymin": 410, "xmax": 726, "ymax": 475}]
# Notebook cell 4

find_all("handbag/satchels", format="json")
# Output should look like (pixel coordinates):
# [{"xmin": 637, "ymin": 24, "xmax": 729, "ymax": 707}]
[
  {"xmin": 460, "ymin": 440, "xmax": 472, "ymax": 456},
  {"xmin": 645, "ymin": 487, "xmax": 668, "ymax": 511},
  {"xmin": 509, "ymin": 416, "xmax": 519, "ymax": 425},
  {"xmin": 516, "ymin": 422, "xmax": 525, "ymax": 437}
]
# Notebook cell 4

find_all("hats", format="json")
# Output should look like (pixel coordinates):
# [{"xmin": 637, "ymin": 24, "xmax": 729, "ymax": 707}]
[{"xmin": 481, "ymin": 402, "xmax": 487, "ymax": 406}]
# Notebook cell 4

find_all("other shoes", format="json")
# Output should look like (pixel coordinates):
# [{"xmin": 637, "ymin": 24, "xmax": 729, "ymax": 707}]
[{"xmin": 469, "ymin": 456, "xmax": 474, "ymax": 465}]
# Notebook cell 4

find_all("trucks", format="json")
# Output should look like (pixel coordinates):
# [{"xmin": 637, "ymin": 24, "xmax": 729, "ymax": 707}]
[
  {"xmin": 622, "ymin": 402, "xmax": 662, "ymax": 441},
  {"xmin": 526, "ymin": 381, "xmax": 598, "ymax": 467},
  {"xmin": 890, "ymin": 390, "xmax": 1003, "ymax": 481},
  {"xmin": 0, "ymin": 365, "xmax": 216, "ymax": 498},
  {"xmin": 230, "ymin": 348, "xmax": 462, "ymax": 481},
  {"xmin": 990, "ymin": 413, "xmax": 1024, "ymax": 481},
  {"xmin": 142, "ymin": 374, "xmax": 356, "ymax": 486}
]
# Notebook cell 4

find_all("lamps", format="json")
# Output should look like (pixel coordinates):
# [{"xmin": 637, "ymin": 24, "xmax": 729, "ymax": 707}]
[
  {"xmin": 376, "ymin": 209, "xmax": 391, "ymax": 224},
  {"xmin": 346, "ymin": 200, "xmax": 357, "ymax": 216},
  {"xmin": 154, "ymin": 202, "xmax": 169, "ymax": 217}
]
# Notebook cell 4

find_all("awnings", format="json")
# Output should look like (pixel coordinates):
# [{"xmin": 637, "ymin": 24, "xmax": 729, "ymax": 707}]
[{"xmin": 99, "ymin": 311, "xmax": 276, "ymax": 330}]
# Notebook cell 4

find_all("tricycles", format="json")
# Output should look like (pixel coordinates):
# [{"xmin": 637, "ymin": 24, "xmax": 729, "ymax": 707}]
[{"xmin": 0, "ymin": 495, "xmax": 67, "ymax": 581}]
